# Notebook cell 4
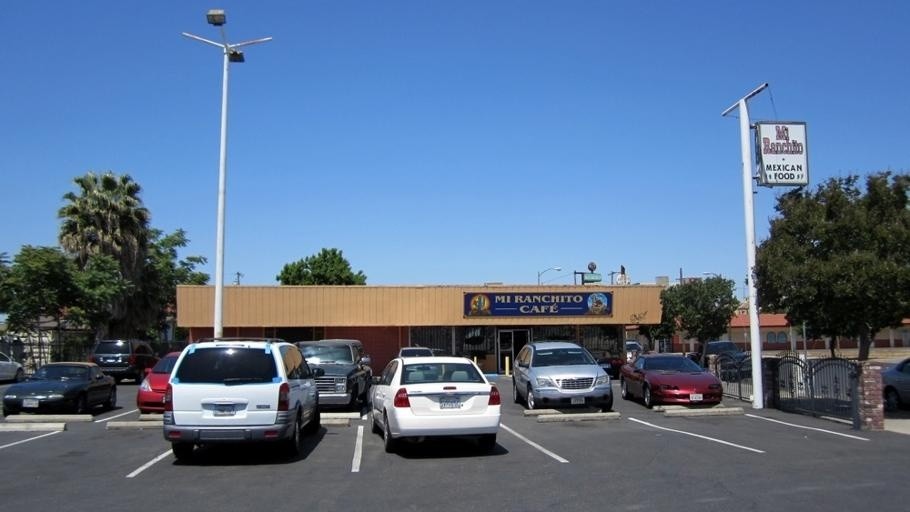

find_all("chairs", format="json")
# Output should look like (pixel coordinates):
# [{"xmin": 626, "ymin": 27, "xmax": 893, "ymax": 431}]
[
  {"xmin": 424, "ymin": 370, "xmax": 439, "ymax": 381},
  {"xmin": 450, "ymin": 370, "xmax": 470, "ymax": 382},
  {"xmin": 407, "ymin": 371, "xmax": 425, "ymax": 383}
]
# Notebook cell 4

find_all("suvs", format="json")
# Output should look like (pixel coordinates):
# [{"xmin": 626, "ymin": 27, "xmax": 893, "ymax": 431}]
[{"xmin": 512, "ymin": 341, "xmax": 613, "ymax": 414}]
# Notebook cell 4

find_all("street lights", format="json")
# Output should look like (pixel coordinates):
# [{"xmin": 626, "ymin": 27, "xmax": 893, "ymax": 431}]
[
  {"xmin": 537, "ymin": 267, "xmax": 562, "ymax": 286},
  {"xmin": 182, "ymin": 7, "xmax": 272, "ymax": 340},
  {"xmin": 703, "ymin": 272, "xmax": 719, "ymax": 279}
]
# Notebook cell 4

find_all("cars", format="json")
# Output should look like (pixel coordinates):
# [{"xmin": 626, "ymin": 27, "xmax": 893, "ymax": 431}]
[
  {"xmin": 592, "ymin": 339, "xmax": 751, "ymax": 412},
  {"xmin": 881, "ymin": 355, "xmax": 909, "ymax": 417}
]
